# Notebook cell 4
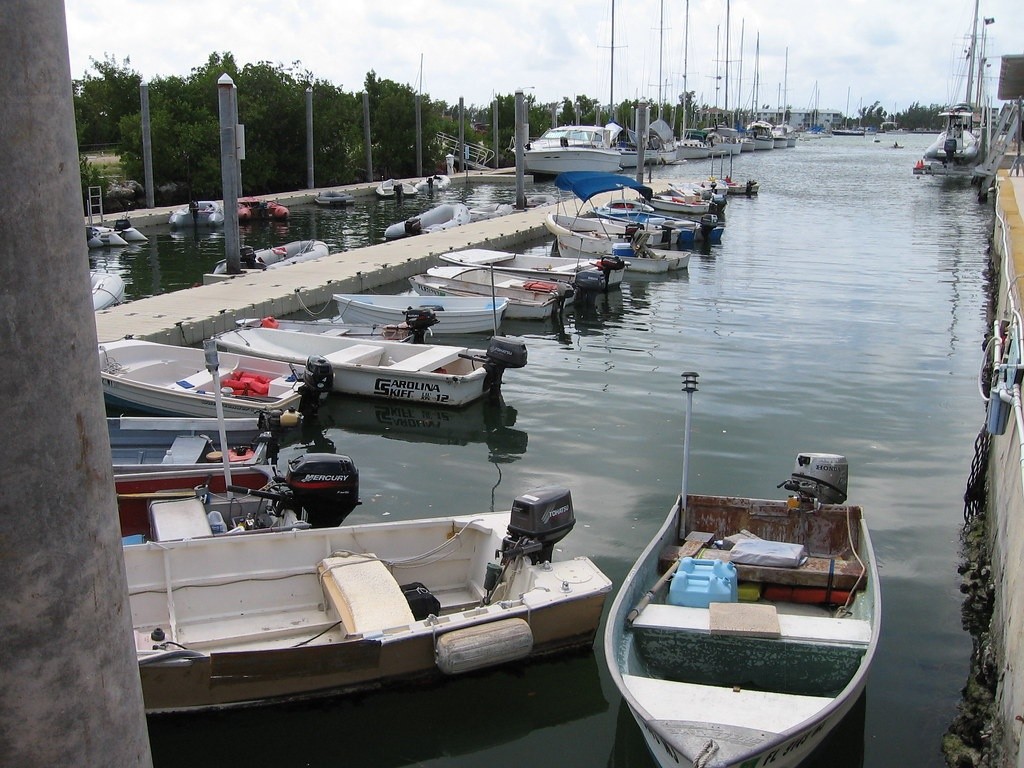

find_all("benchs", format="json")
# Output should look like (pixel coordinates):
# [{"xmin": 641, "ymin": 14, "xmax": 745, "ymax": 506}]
[
  {"xmin": 169, "ymin": 365, "xmax": 232, "ymax": 392},
  {"xmin": 318, "ymin": 552, "xmax": 415, "ymax": 641},
  {"xmin": 659, "ymin": 546, "xmax": 868, "ymax": 592},
  {"xmin": 391, "ymin": 347, "xmax": 467, "ymax": 371},
  {"xmin": 160, "ymin": 435, "xmax": 207, "ymax": 464},
  {"xmin": 324, "ymin": 344, "xmax": 385, "ymax": 366},
  {"xmin": 632, "ymin": 603, "xmax": 871, "ymax": 698}
]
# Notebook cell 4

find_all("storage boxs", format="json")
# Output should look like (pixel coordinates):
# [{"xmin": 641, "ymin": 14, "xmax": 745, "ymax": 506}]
[{"xmin": 612, "ymin": 242, "xmax": 639, "ymax": 258}]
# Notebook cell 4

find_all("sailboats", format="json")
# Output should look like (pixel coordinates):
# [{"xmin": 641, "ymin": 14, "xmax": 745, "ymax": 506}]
[
  {"xmin": 798, "ymin": 80, "xmax": 877, "ymax": 136},
  {"xmin": 596, "ymin": 1, "xmax": 796, "ymax": 167},
  {"xmin": 919, "ymin": 0, "xmax": 985, "ymax": 162}
]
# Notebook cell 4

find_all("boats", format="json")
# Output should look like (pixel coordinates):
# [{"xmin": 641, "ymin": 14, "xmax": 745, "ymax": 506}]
[
  {"xmin": 85, "ymin": 228, "xmax": 129, "ymax": 245},
  {"xmin": 238, "ymin": 303, "xmax": 444, "ymax": 345},
  {"xmin": 384, "ymin": 196, "xmax": 556, "ymax": 239},
  {"xmin": 410, "ymin": 267, "xmax": 574, "ymax": 317},
  {"xmin": 213, "ymin": 240, "xmax": 328, "ymax": 274},
  {"xmin": 238, "ymin": 196, "xmax": 289, "ymax": 220},
  {"xmin": 415, "ymin": 174, "xmax": 450, "ymax": 193},
  {"xmin": 170, "ymin": 200, "xmax": 223, "ymax": 229},
  {"xmin": 523, "ymin": 125, "xmax": 623, "ymax": 174},
  {"xmin": 98, "ymin": 337, "xmax": 334, "ymax": 428},
  {"xmin": 440, "ymin": 249, "xmax": 628, "ymax": 294},
  {"xmin": 315, "ymin": 191, "xmax": 355, "ymax": 205},
  {"xmin": 334, "ymin": 295, "xmax": 510, "ymax": 335},
  {"xmin": 124, "ymin": 491, "xmax": 612, "ymax": 720},
  {"xmin": 90, "ymin": 272, "xmax": 125, "ymax": 311},
  {"xmin": 726, "ymin": 177, "xmax": 759, "ymax": 195},
  {"xmin": 891, "ymin": 142, "xmax": 904, "ymax": 148},
  {"xmin": 603, "ymin": 369, "xmax": 882, "ymax": 768},
  {"xmin": 874, "ymin": 137, "xmax": 880, "ymax": 141},
  {"xmin": 213, "ymin": 326, "xmax": 525, "ymax": 412},
  {"xmin": 107, "ymin": 407, "xmax": 362, "ymax": 543},
  {"xmin": 376, "ymin": 175, "xmax": 418, "ymax": 197},
  {"xmin": 105, "ymin": 220, "xmax": 147, "ymax": 241},
  {"xmin": 544, "ymin": 181, "xmax": 727, "ymax": 274}
]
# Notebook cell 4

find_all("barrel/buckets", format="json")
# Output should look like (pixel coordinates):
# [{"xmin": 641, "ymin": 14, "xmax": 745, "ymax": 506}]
[
  {"xmin": 668, "ymin": 572, "xmax": 732, "ymax": 609},
  {"xmin": 677, "ymin": 556, "xmax": 739, "ymax": 603}
]
